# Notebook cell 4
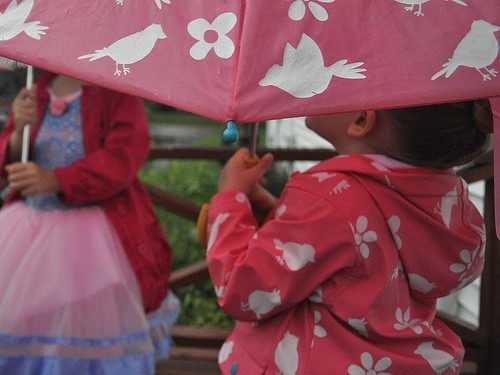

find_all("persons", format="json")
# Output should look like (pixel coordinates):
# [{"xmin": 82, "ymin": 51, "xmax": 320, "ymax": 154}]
[
  {"xmin": 198, "ymin": 98, "xmax": 493, "ymax": 375},
  {"xmin": 0, "ymin": 71, "xmax": 181, "ymax": 375}
]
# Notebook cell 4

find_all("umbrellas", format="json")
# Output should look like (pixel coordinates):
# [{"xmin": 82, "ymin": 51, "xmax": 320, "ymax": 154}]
[{"xmin": 0, "ymin": 0, "xmax": 500, "ymax": 165}]
